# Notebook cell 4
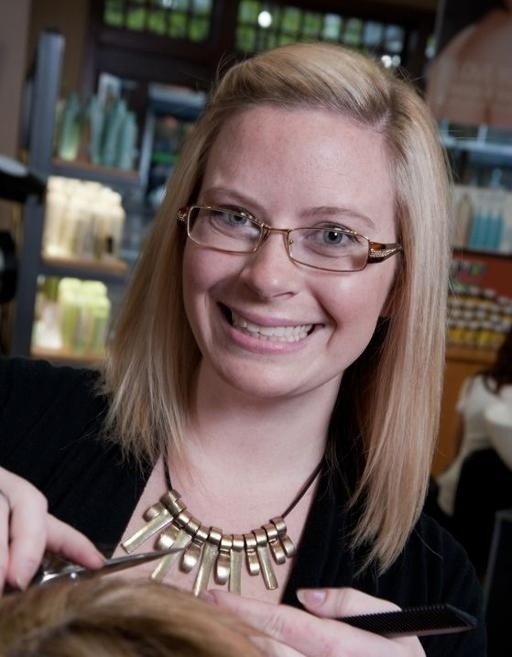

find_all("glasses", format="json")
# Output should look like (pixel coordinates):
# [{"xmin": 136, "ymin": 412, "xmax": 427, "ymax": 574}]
[{"xmin": 175, "ymin": 198, "xmax": 404, "ymax": 275}]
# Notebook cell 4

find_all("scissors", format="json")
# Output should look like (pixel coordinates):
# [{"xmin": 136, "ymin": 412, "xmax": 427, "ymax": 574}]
[{"xmin": 4, "ymin": 548, "xmax": 186, "ymax": 597}]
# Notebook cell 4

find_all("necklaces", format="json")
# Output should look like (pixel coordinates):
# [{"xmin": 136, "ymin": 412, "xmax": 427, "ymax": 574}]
[{"xmin": 116, "ymin": 441, "xmax": 327, "ymax": 601}]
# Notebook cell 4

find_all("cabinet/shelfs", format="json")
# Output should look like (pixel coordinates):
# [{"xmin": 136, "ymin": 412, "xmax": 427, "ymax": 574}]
[
  {"xmin": 436, "ymin": 245, "xmax": 510, "ymax": 477},
  {"xmin": 26, "ymin": 150, "xmax": 148, "ymax": 370}
]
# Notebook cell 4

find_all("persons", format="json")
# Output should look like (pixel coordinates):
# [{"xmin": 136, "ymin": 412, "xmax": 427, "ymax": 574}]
[
  {"xmin": 0, "ymin": 570, "xmax": 280, "ymax": 655},
  {"xmin": 423, "ymin": 319, "xmax": 512, "ymax": 534},
  {"xmin": 0, "ymin": 36, "xmax": 491, "ymax": 655}
]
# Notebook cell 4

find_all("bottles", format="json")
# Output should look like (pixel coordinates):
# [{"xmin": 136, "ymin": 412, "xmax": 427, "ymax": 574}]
[
  {"xmin": 453, "ymin": 193, "xmax": 504, "ymax": 251},
  {"xmin": 147, "ymin": 108, "xmax": 200, "ymax": 216},
  {"xmin": 54, "ymin": 275, "xmax": 112, "ymax": 360},
  {"xmin": 44, "ymin": 174, "xmax": 128, "ymax": 262},
  {"xmin": 54, "ymin": 88, "xmax": 141, "ymax": 170},
  {"xmin": 444, "ymin": 278, "xmax": 511, "ymax": 353}
]
